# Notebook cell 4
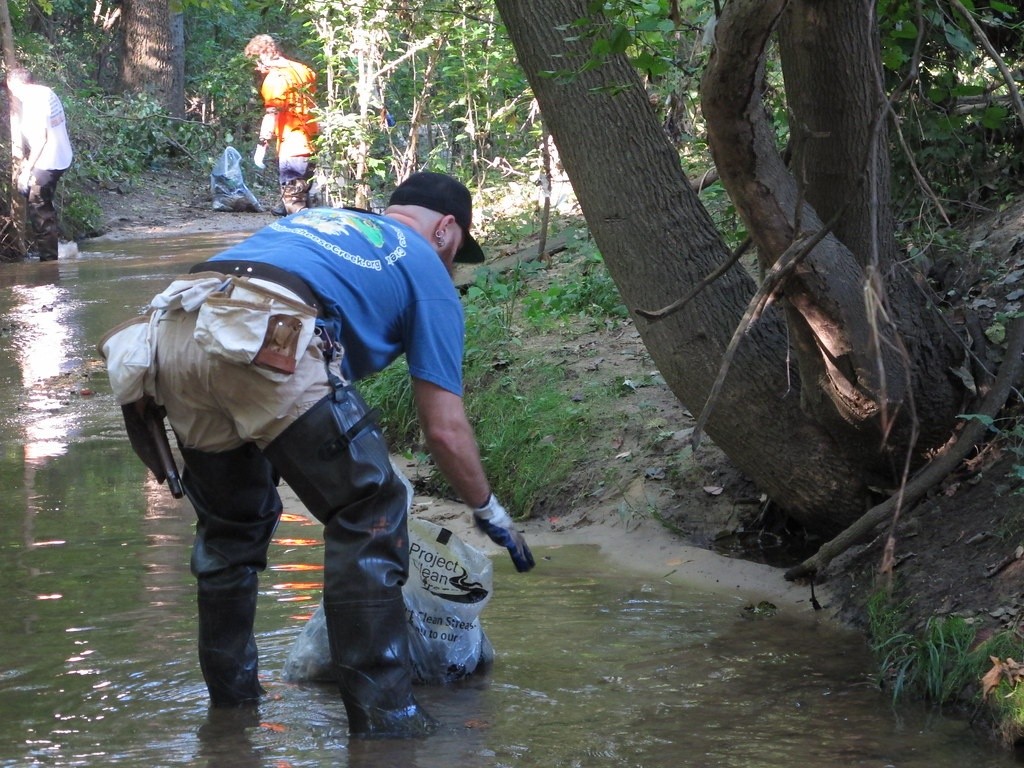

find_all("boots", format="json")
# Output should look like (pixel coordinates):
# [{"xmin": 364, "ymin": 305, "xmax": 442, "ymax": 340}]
[
  {"xmin": 195, "ymin": 575, "xmax": 268, "ymax": 703},
  {"xmin": 326, "ymin": 591, "xmax": 445, "ymax": 738}
]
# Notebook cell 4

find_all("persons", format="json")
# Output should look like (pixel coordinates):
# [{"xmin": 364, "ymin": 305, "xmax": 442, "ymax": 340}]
[
  {"xmin": 5, "ymin": 66, "xmax": 73, "ymax": 262},
  {"xmin": 96, "ymin": 171, "xmax": 538, "ymax": 742},
  {"xmin": 246, "ymin": 34, "xmax": 317, "ymax": 215}
]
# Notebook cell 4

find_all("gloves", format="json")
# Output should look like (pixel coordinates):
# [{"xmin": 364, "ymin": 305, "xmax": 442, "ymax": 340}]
[
  {"xmin": 475, "ymin": 493, "xmax": 535, "ymax": 572},
  {"xmin": 253, "ymin": 142, "xmax": 267, "ymax": 170},
  {"xmin": 19, "ymin": 166, "xmax": 33, "ymax": 197}
]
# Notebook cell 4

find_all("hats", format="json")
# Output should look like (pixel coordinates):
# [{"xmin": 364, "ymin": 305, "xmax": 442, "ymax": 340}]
[{"xmin": 390, "ymin": 172, "xmax": 484, "ymax": 265}]
